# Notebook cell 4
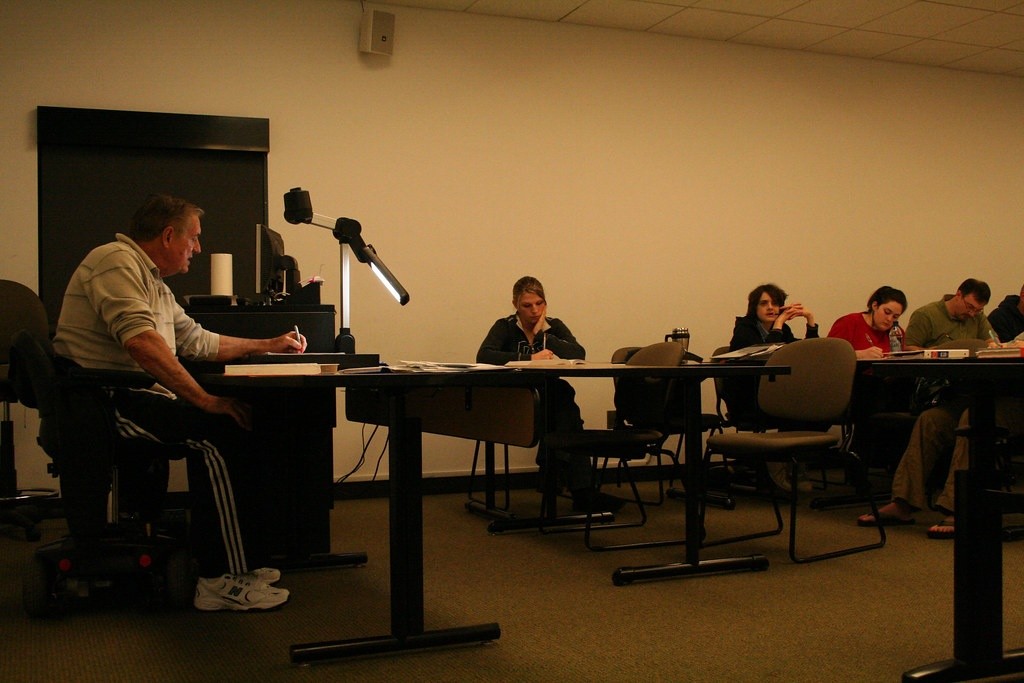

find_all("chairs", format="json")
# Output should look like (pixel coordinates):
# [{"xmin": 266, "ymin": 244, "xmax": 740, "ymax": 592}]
[
  {"xmin": 469, "ymin": 338, "xmax": 1023, "ymax": 564},
  {"xmin": 0, "ymin": 281, "xmax": 189, "ymax": 607}
]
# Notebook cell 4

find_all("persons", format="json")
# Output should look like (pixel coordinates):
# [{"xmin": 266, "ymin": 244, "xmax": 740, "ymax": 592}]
[
  {"xmin": 49, "ymin": 190, "xmax": 306, "ymax": 614},
  {"xmin": 856, "ymin": 332, "xmax": 1024, "ymax": 538},
  {"xmin": 828, "ymin": 284, "xmax": 907, "ymax": 491},
  {"xmin": 476, "ymin": 274, "xmax": 605, "ymax": 511},
  {"xmin": 727, "ymin": 284, "xmax": 832, "ymax": 497},
  {"xmin": 987, "ymin": 284, "xmax": 1024, "ymax": 343},
  {"xmin": 902, "ymin": 279, "xmax": 999, "ymax": 421}
]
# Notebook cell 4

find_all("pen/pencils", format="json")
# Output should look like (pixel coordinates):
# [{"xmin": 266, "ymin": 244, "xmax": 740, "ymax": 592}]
[
  {"xmin": 543, "ymin": 332, "xmax": 546, "ymax": 350},
  {"xmin": 293, "ymin": 325, "xmax": 303, "ymax": 353},
  {"xmin": 774, "ymin": 313, "xmax": 782, "ymax": 315},
  {"xmin": 945, "ymin": 334, "xmax": 954, "ymax": 342},
  {"xmin": 865, "ymin": 332, "xmax": 873, "ymax": 347}
]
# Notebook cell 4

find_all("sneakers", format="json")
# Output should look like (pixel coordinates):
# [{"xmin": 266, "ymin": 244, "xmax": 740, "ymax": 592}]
[
  {"xmin": 251, "ymin": 567, "xmax": 282, "ymax": 586},
  {"xmin": 194, "ymin": 573, "xmax": 292, "ymax": 612}
]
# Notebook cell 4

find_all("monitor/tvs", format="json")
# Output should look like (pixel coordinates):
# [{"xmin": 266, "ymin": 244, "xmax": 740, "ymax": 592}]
[{"xmin": 255, "ymin": 224, "xmax": 300, "ymax": 304}]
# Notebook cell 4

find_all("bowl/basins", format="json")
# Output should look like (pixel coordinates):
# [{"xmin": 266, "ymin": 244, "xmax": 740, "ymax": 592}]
[{"xmin": 190, "ymin": 297, "xmax": 232, "ymax": 314}]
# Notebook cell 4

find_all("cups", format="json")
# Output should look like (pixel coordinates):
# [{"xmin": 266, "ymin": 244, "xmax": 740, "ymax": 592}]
[{"xmin": 665, "ymin": 327, "xmax": 690, "ymax": 363}]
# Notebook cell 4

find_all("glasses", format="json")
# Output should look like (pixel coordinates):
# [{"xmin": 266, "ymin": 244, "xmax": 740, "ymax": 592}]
[{"xmin": 961, "ymin": 293, "xmax": 984, "ymax": 315}]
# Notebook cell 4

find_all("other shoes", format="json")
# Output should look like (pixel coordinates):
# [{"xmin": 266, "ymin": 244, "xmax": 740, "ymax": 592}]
[
  {"xmin": 796, "ymin": 463, "xmax": 813, "ymax": 492},
  {"xmin": 767, "ymin": 460, "xmax": 793, "ymax": 492}
]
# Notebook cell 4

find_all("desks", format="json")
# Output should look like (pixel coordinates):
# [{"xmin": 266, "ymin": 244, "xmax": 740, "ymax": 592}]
[
  {"xmin": 718, "ymin": 349, "xmax": 1024, "ymax": 683},
  {"xmin": 191, "ymin": 362, "xmax": 540, "ymax": 657},
  {"xmin": 504, "ymin": 360, "xmax": 795, "ymax": 586}
]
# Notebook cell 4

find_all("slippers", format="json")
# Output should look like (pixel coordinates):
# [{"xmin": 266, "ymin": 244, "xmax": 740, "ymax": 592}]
[
  {"xmin": 927, "ymin": 521, "xmax": 955, "ymax": 538},
  {"xmin": 857, "ymin": 509, "xmax": 916, "ymax": 525}
]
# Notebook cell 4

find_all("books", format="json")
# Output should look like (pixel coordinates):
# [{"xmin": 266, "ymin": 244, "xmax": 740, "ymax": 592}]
[
  {"xmin": 223, "ymin": 364, "xmax": 340, "ymax": 374},
  {"xmin": 506, "ymin": 354, "xmax": 611, "ymax": 367},
  {"xmin": 974, "ymin": 346, "xmax": 1024, "ymax": 358}
]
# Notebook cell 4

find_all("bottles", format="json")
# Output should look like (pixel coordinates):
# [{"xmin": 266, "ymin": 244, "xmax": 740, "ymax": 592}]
[
  {"xmin": 889, "ymin": 321, "xmax": 902, "ymax": 352},
  {"xmin": 210, "ymin": 253, "xmax": 233, "ymax": 296}
]
